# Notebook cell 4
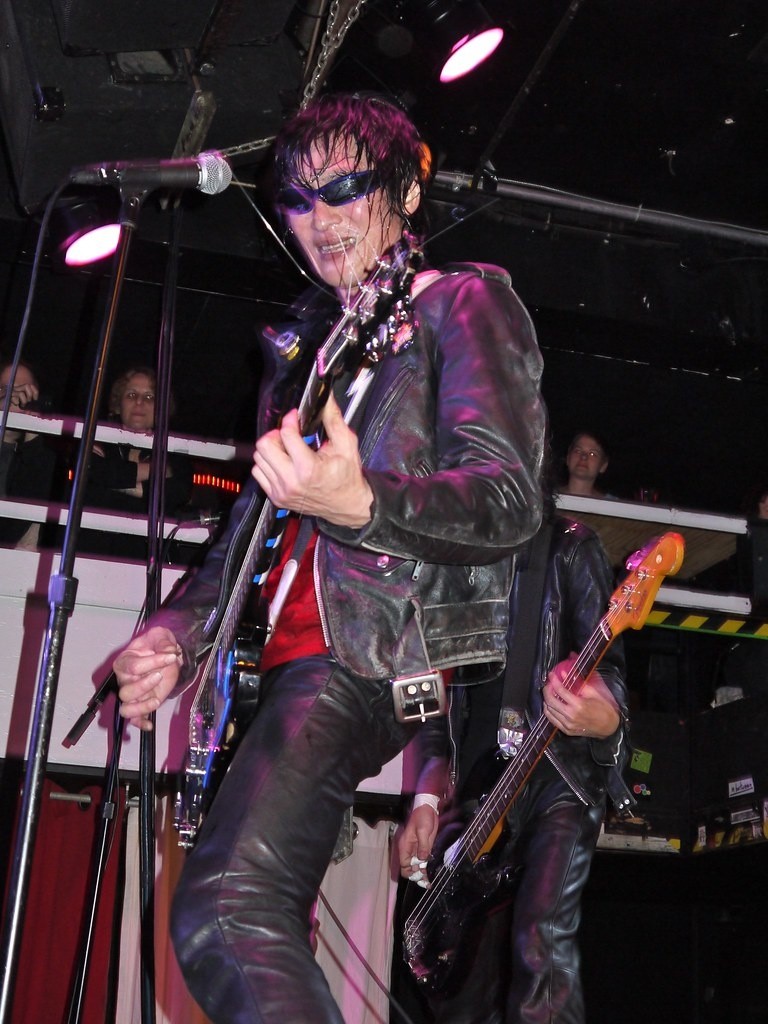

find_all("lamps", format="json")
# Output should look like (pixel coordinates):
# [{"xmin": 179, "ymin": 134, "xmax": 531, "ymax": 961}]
[
  {"xmin": 38, "ymin": 185, "xmax": 122, "ymax": 267},
  {"xmin": 402, "ymin": 0, "xmax": 504, "ymax": 84}
]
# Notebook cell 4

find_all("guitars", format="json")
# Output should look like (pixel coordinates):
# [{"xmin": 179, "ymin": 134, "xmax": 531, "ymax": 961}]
[
  {"xmin": 395, "ymin": 530, "xmax": 687, "ymax": 1000},
  {"xmin": 173, "ymin": 227, "xmax": 432, "ymax": 853}
]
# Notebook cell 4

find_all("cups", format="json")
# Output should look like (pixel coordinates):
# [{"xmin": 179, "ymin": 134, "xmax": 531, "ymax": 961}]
[{"xmin": 635, "ymin": 488, "xmax": 660, "ymax": 504}]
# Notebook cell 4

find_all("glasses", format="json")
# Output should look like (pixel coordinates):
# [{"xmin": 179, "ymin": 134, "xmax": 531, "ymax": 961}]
[
  {"xmin": 122, "ymin": 392, "xmax": 155, "ymax": 403},
  {"xmin": 276, "ymin": 168, "xmax": 383, "ymax": 214}
]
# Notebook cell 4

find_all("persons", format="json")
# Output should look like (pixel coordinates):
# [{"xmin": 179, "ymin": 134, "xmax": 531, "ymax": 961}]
[
  {"xmin": 555, "ymin": 433, "xmax": 620, "ymax": 499},
  {"xmin": 0, "ymin": 362, "xmax": 203, "ymax": 569},
  {"xmin": 393, "ymin": 466, "xmax": 630, "ymax": 1024},
  {"xmin": 112, "ymin": 100, "xmax": 548, "ymax": 1024}
]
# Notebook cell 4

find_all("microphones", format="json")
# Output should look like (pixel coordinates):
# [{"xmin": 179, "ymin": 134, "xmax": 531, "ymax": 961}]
[{"xmin": 72, "ymin": 153, "xmax": 232, "ymax": 194}]
